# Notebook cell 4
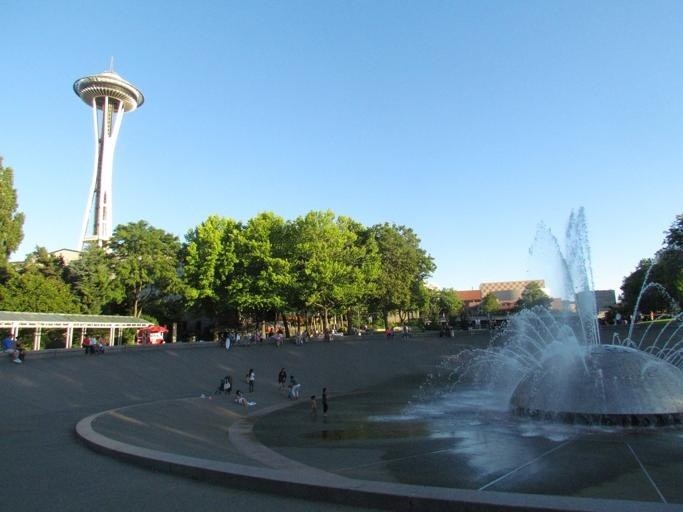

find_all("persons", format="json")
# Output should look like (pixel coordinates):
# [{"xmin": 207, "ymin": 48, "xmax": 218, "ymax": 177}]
[
  {"xmin": 216, "ymin": 364, "xmax": 302, "ymax": 407},
  {"xmin": 310, "ymin": 396, "xmax": 318, "ymax": 416},
  {"xmin": 82, "ymin": 334, "xmax": 105, "ymax": 355},
  {"xmin": 323, "ymin": 387, "xmax": 328, "ymax": 416},
  {"xmin": 3, "ymin": 334, "xmax": 26, "ymax": 363},
  {"xmin": 218, "ymin": 327, "xmax": 408, "ymax": 349}
]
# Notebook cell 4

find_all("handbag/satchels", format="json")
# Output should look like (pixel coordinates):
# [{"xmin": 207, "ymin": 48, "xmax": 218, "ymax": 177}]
[
  {"xmin": 246, "ymin": 378, "xmax": 249, "ymax": 384},
  {"xmin": 224, "ymin": 383, "xmax": 231, "ymax": 390}
]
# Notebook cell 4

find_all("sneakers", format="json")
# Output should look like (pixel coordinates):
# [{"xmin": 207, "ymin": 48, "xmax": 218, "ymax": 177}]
[{"xmin": 14, "ymin": 359, "xmax": 21, "ymax": 363}]
